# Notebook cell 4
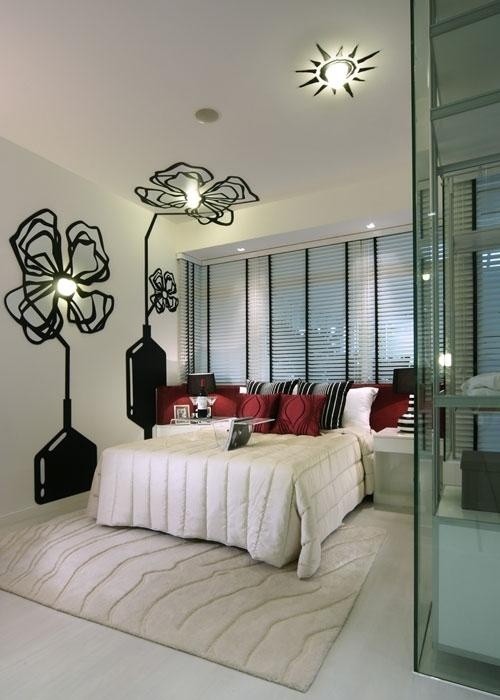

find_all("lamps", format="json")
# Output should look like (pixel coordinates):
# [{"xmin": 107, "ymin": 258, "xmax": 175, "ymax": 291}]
[
  {"xmin": 126, "ymin": 162, "xmax": 260, "ymax": 441},
  {"xmin": 391, "ymin": 368, "xmax": 417, "ymax": 432},
  {"xmin": 295, "ymin": 42, "xmax": 381, "ymax": 98},
  {"xmin": 4, "ymin": 208, "xmax": 114, "ymax": 504},
  {"xmin": 185, "ymin": 372, "xmax": 217, "ymax": 417}
]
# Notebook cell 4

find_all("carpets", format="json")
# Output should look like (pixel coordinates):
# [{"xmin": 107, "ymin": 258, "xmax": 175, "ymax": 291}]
[{"xmin": 0, "ymin": 510, "xmax": 387, "ymax": 692}]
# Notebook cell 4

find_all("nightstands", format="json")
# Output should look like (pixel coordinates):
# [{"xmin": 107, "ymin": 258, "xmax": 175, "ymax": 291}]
[
  {"xmin": 371, "ymin": 427, "xmax": 444, "ymax": 517},
  {"xmin": 152, "ymin": 416, "xmax": 228, "ymax": 437}
]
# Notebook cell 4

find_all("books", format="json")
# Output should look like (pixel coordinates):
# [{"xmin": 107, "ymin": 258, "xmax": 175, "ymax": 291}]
[{"xmin": 224, "ymin": 418, "xmax": 253, "ymax": 451}]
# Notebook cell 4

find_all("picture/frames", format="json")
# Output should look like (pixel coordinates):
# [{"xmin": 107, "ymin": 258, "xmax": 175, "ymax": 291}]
[{"xmin": 174, "ymin": 405, "xmax": 190, "ymax": 424}]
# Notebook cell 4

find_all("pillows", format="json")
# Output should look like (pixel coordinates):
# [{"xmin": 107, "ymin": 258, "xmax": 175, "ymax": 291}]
[
  {"xmin": 246, "ymin": 379, "xmax": 299, "ymax": 394},
  {"xmin": 341, "ymin": 386, "xmax": 380, "ymax": 434},
  {"xmin": 271, "ymin": 393, "xmax": 325, "ymax": 437},
  {"xmin": 236, "ymin": 394, "xmax": 279, "ymax": 433},
  {"xmin": 298, "ymin": 379, "xmax": 354, "ymax": 430}
]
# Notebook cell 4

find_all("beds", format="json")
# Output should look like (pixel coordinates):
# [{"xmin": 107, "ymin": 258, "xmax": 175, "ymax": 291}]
[{"xmin": 89, "ymin": 383, "xmax": 408, "ymax": 581}]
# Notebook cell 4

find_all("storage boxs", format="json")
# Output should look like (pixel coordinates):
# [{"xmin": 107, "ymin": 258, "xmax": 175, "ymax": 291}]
[{"xmin": 459, "ymin": 450, "xmax": 500, "ymax": 513}]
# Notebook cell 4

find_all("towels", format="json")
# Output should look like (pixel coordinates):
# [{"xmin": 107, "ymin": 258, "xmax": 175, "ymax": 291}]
[{"xmin": 460, "ymin": 372, "xmax": 500, "ymax": 397}]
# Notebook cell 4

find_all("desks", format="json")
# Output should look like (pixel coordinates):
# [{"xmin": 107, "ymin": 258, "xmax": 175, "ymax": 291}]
[{"xmin": 437, "ymin": 486, "xmax": 500, "ymax": 666}]
